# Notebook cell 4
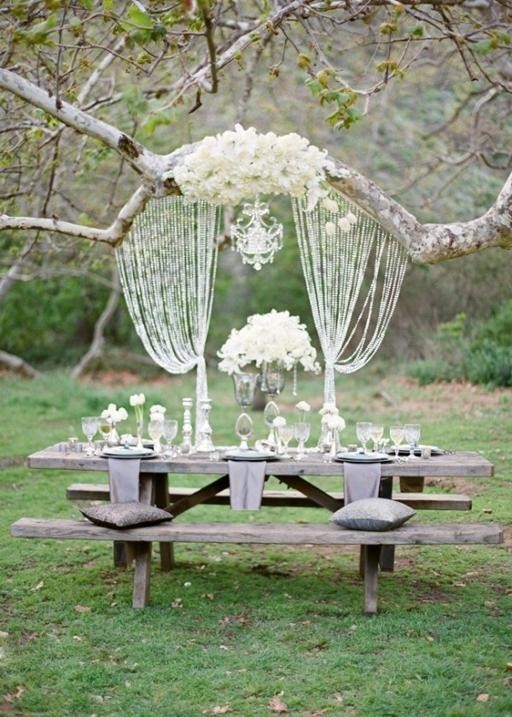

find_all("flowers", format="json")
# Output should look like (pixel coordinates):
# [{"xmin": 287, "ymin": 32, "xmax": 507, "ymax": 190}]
[
  {"xmin": 173, "ymin": 122, "xmax": 357, "ymax": 238},
  {"xmin": 101, "ymin": 308, "xmax": 346, "ymax": 431}
]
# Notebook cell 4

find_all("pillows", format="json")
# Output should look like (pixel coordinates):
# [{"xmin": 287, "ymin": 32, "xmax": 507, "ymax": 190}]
[
  {"xmin": 81, "ymin": 500, "xmax": 176, "ymax": 529},
  {"xmin": 329, "ymin": 498, "xmax": 417, "ymax": 532}
]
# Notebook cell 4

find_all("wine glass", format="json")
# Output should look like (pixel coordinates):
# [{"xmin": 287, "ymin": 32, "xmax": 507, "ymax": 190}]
[
  {"xmin": 148, "ymin": 420, "xmax": 178, "ymax": 456},
  {"xmin": 81, "ymin": 416, "xmax": 111, "ymax": 456},
  {"xmin": 356, "ymin": 422, "xmax": 420, "ymax": 459},
  {"xmin": 278, "ymin": 423, "xmax": 310, "ymax": 461}
]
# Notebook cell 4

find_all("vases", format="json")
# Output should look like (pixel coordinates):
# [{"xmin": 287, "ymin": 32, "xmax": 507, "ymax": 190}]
[
  {"xmin": 275, "ymin": 431, "xmax": 285, "ymax": 456},
  {"xmin": 327, "ymin": 429, "xmax": 340, "ymax": 455},
  {"xmin": 107, "ymin": 423, "xmax": 119, "ymax": 447},
  {"xmin": 135, "ymin": 421, "xmax": 145, "ymax": 451}
]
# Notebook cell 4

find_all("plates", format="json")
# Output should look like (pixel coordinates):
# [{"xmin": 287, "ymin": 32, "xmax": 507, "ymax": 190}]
[
  {"xmin": 221, "ymin": 451, "xmax": 276, "ymax": 462},
  {"xmin": 333, "ymin": 453, "xmax": 391, "ymax": 464},
  {"xmin": 100, "ymin": 446, "xmax": 159, "ymax": 460},
  {"xmin": 390, "ymin": 445, "xmax": 443, "ymax": 456}
]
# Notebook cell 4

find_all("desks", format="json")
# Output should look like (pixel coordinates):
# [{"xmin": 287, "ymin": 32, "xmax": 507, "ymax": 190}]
[{"xmin": 27, "ymin": 440, "xmax": 493, "ymax": 615}]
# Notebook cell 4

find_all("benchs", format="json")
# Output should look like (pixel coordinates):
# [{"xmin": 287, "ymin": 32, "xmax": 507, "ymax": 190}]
[
  {"xmin": 10, "ymin": 518, "xmax": 504, "ymax": 615},
  {"xmin": 66, "ymin": 483, "xmax": 473, "ymax": 570}
]
